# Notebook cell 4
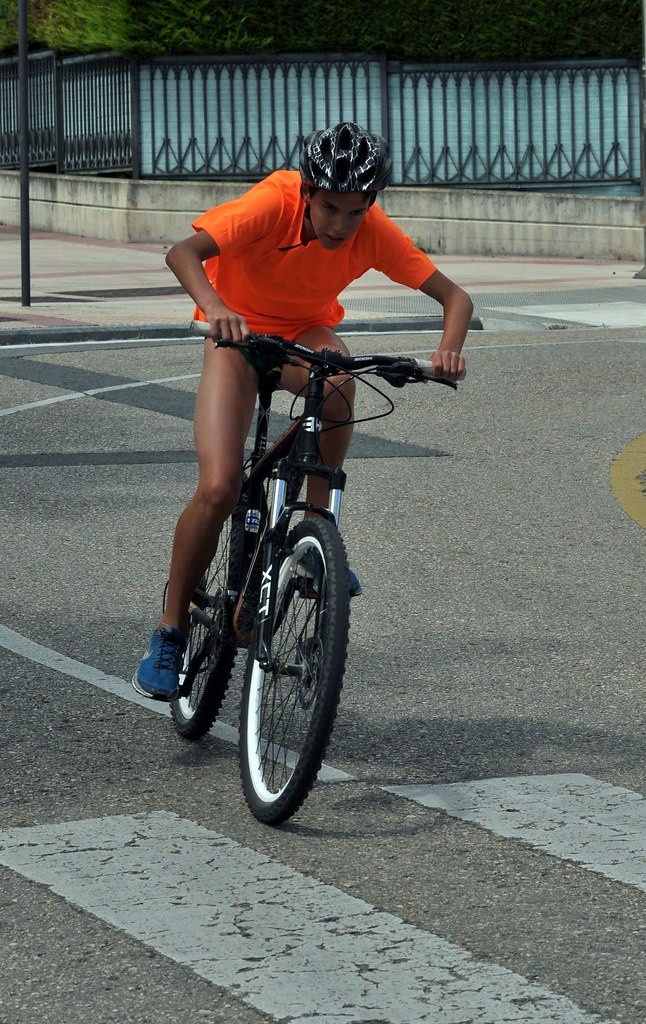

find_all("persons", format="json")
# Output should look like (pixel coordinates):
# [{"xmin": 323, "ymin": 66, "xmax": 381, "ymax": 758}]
[{"xmin": 132, "ymin": 121, "xmax": 473, "ymax": 703}]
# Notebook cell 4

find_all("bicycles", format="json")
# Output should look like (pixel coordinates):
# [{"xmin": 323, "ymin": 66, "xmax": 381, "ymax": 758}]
[{"xmin": 159, "ymin": 318, "xmax": 465, "ymax": 828}]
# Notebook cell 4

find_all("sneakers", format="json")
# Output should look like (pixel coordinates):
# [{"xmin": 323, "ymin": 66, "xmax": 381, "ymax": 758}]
[
  {"xmin": 297, "ymin": 545, "xmax": 362, "ymax": 596},
  {"xmin": 132, "ymin": 623, "xmax": 189, "ymax": 701}
]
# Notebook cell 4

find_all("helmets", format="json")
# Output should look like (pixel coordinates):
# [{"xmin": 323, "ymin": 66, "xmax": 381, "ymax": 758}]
[{"xmin": 299, "ymin": 122, "xmax": 392, "ymax": 191}]
